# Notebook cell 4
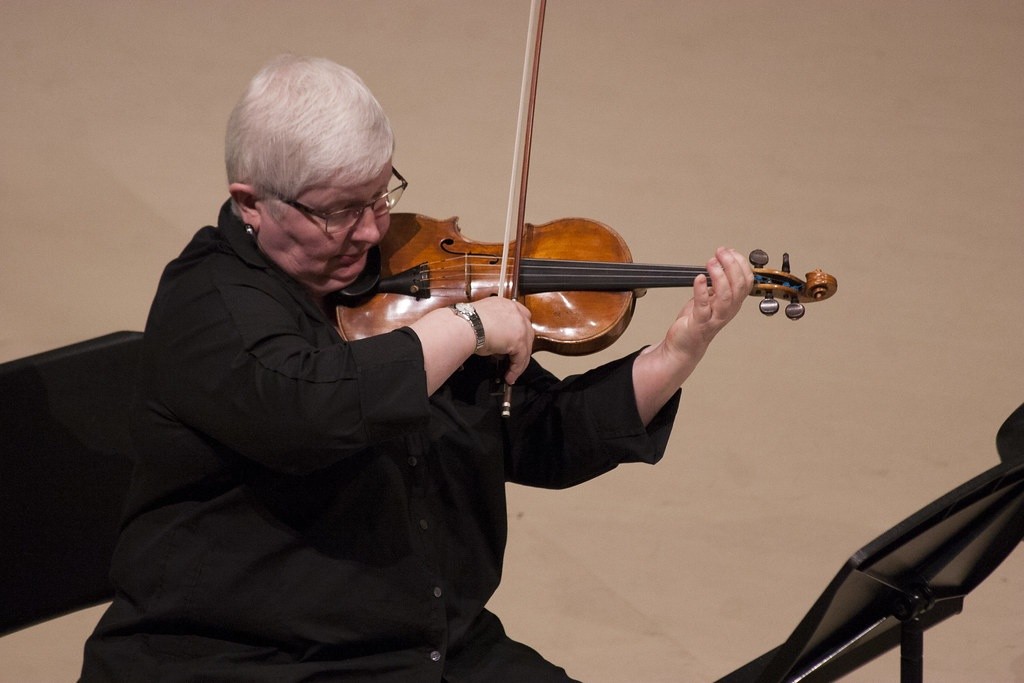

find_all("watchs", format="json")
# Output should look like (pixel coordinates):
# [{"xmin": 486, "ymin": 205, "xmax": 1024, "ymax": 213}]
[{"xmin": 448, "ymin": 302, "xmax": 486, "ymax": 353}]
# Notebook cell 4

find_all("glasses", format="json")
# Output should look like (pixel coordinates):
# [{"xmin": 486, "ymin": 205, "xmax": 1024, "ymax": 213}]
[{"xmin": 258, "ymin": 165, "xmax": 408, "ymax": 235}]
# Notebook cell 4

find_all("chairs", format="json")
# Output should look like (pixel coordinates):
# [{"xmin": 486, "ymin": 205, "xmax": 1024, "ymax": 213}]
[{"xmin": 0, "ymin": 330, "xmax": 144, "ymax": 638}]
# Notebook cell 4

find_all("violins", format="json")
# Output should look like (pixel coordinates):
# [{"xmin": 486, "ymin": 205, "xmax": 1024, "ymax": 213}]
[{"xmin": 335, "ymin": 212, "xmax": 838, "ymax": 358}]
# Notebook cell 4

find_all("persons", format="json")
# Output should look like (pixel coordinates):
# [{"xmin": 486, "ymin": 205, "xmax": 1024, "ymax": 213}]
[{"xmin": 77, "ymin": 54, "xmax": 754, "ymax": 683}]
